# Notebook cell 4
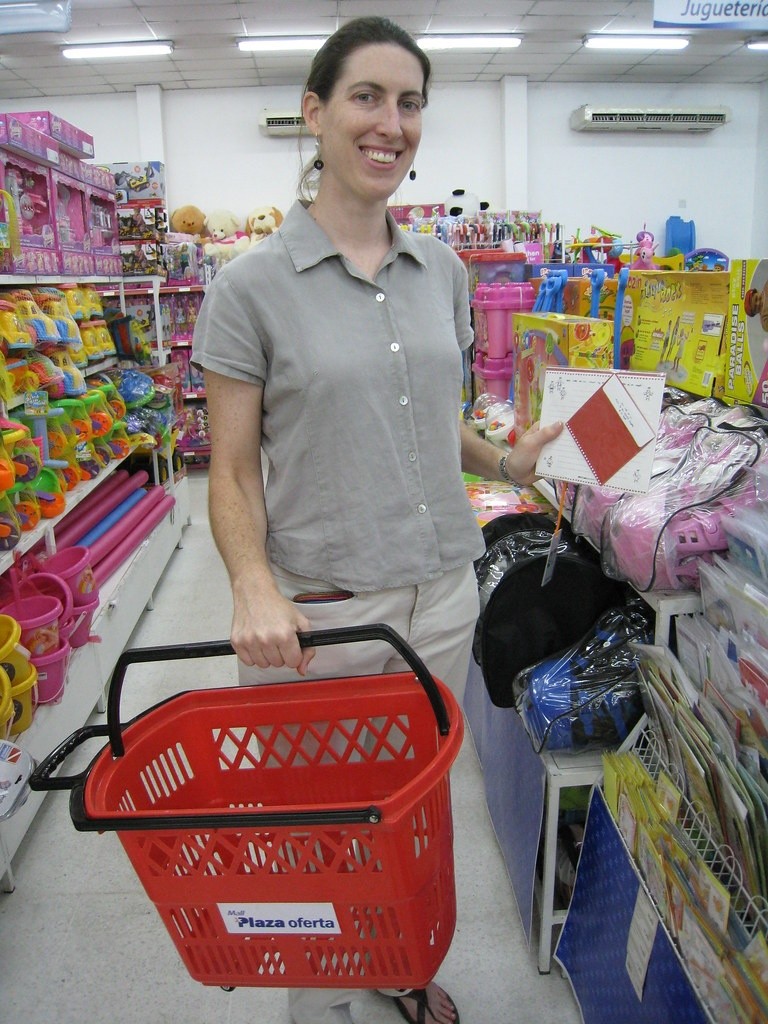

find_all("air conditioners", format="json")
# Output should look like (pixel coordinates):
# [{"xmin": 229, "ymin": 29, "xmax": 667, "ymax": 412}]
[
  {"xmin": 261, "ymin": 109, "xmax": 316, "ymax": 137},
  {"xmin": 571, "ymin": 106, "xmax": 732, "ymax": 134}
]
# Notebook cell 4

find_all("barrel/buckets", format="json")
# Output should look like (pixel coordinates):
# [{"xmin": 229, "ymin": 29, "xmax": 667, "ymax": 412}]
[{"xmin": 0, "ymin": 546, "xmax": 100, "ymax": 740}]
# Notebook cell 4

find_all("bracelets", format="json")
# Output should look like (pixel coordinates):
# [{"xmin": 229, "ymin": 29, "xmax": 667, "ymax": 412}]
[{"xmin": 498, "ymin": 451, "xmax": 526, "ymax": 490}]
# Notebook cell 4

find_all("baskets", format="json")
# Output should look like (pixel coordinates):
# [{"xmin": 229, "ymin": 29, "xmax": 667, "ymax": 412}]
[{"xmin": 26, "ymin": 624, "xmax": 464, "ymax": 989}]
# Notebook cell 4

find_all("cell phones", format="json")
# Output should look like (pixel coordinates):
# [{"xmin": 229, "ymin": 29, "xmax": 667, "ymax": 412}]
[{"xmin": 292, "ymin": 591, "xmax": 353, "ymax": 605}]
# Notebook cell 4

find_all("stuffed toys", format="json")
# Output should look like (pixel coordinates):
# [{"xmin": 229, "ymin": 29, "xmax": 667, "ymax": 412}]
[{"xmin": 169, "ymin": 205, "xmax": 283, "ymax": 271}]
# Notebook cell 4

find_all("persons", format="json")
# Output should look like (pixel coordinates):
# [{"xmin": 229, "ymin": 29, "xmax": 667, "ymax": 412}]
[{"xmin": 191, "ymin": 20, "xmax": 568, "ymax": 1024}]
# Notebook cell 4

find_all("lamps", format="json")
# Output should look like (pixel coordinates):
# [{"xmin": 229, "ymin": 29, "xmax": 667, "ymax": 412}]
[
  {"xmin": 414, "ymin": 32, "xmax": 524, "ymax": 48},
  {"xmin": 54, "ymin": 41, "xmax": 177, "ymax": 58},
  {"xmin": 580, "ymin": 35, "xmax": 694, "ymax": 51},
  {"xmin": 747, "ymin": 36, "xmax": 768, "ymax": 50},
  {"xmin": 234, "ymin": 35, "xmax": 334, "ymax": 50}
]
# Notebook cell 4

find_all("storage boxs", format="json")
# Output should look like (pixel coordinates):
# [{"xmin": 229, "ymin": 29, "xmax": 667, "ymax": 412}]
[{"xmin": 0, "ymin": 106, "xmax": 768, "ymax": 452}]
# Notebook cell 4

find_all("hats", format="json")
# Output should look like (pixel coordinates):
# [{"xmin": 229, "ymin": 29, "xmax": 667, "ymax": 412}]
[{"xmin": 744, "ymin": 288, "xmax": 757, "ymax": 317}]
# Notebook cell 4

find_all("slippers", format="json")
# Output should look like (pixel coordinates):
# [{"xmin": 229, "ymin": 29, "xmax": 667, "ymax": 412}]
[{"xmin": 393, "ymin": 981, "xmax": 460, "ymax": 1024}]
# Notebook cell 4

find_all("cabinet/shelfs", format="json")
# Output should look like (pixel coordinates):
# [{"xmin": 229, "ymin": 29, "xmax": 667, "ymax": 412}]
[
  {"xmin": 467, "ymin": 482, "xmax": 704, "ymax": 977},
  {"xmin": 0, "ymin": 270, "xmax": 180, "ymax": 899},
  {"xmin": 96, "ymin": 283, "xmax": 211, "ymax": 470}
]
